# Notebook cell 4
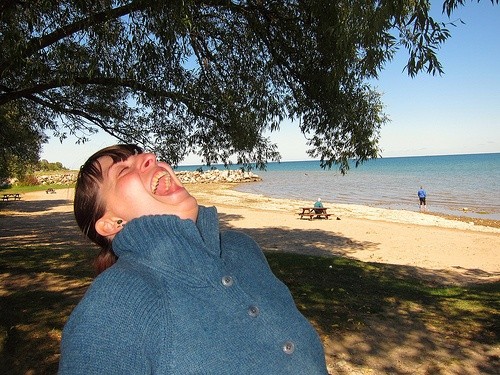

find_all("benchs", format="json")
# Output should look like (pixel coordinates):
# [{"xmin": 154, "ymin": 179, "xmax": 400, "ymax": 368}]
[
  {"xmin": 46, "ymin": 188, "xmax": 56, "ymax": 194},
  {"xmin": 2, "ymin": 194, "xmax": 22, "ymax": 202},
  {"xmin": 299, "ymin": 213, "xmax": 333, "ymax": 215}
]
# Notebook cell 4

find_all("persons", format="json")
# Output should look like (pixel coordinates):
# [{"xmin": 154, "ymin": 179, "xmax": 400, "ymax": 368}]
[
  {"xmin": 418, "ymin": 186, "xmax": 427, "ymax": 212},
  {"xmin": 314, "ymin": 198, "xmax": 324, "ymax": 217},
  {"xmin": 57, "ymin": 144, "xmax": 330, "ymax": 375}
]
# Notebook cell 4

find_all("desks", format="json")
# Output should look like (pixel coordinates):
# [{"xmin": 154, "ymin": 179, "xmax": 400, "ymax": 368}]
[{"xmin": 300, "ymin": 208, "xmax": 328, "ymax": 220}]
